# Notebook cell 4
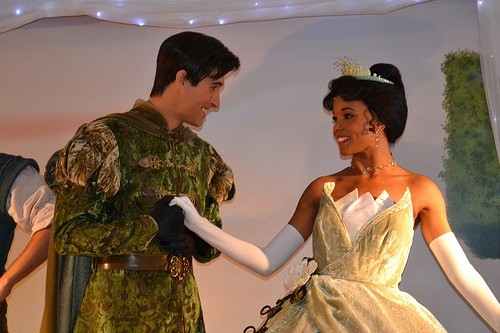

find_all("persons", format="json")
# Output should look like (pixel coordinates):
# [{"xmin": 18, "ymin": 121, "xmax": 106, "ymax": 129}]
[
  {"xmin": 0, "ymin": 151, "xmax": 55, "ymax": 333},
  {"xmin": 172, "ymin": 56, "xmax": 500, "ymax": 332},
  {"xmin": 40, "ymin": 28, "xmax": 240, "ymax": 333}
]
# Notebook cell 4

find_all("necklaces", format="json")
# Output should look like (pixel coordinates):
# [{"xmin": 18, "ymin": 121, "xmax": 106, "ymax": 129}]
[{"xmin": 349, "ymin": 159, "xmax": 397, "ymax": 172}]
